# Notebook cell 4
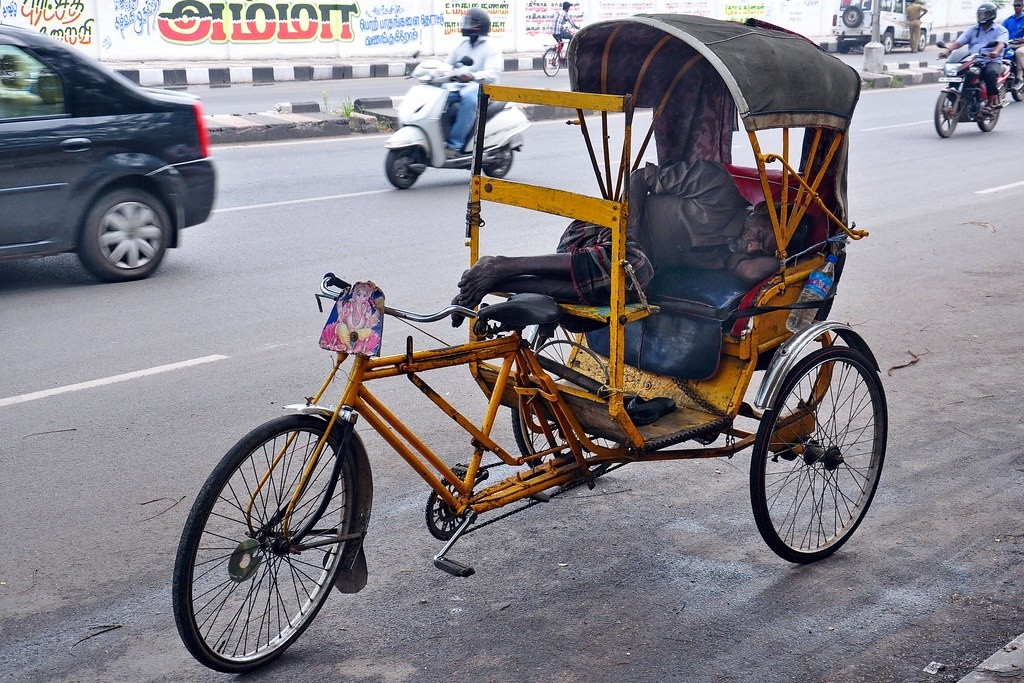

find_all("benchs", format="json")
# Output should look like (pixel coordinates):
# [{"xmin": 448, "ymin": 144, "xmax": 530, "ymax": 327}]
[
  {"xmin": 0, "ymin": 54, "xmax": 67, "ymax": 117},
  {"xmin": 585, "ymin": 163, "xmax": 827, "ymax": 381}
]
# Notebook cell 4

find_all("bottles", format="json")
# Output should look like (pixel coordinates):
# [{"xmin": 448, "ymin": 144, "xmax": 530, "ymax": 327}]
[{"xmin": 785, "ymin": 254, "xmax": 841, "ymax": 333}]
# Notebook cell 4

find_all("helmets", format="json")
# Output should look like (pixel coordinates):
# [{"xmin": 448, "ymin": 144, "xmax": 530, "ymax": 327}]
[
  {"xmin": 461, "ymin": 8, "xmax": 491, "ymax": 37},
  {"xmin": 976, "ymin": 3, "xmax": 997, "ymax": 25}
]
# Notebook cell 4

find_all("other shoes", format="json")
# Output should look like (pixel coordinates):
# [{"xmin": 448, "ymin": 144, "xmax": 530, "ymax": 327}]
[
  {"xmin": 982, "ymin": 106, "xmax": 996, "ymax": 113},
  {"xmin": 1011, "ymin": 78, "xmax": 1024, "ymax": 90},
  {"xmin": 446, "ymin": 149, "xmax": 460, "ymax": 159}
]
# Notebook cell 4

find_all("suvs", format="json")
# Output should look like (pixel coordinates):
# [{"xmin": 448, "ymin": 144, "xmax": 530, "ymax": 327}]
[{"xmin": 831, "ymin": 0, "xmax": 931, "ymax": 54}]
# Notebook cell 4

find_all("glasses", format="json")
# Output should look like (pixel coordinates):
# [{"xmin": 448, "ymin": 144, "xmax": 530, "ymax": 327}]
[{"xmin": 1014, "ymin": 4, "xmax": 1022, "ymax": 7}]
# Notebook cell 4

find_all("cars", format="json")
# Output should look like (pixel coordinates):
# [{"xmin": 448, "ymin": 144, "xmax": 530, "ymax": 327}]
[{"xmin": 0, "ymin": 22, "xmax": 217, "ymax": 284}]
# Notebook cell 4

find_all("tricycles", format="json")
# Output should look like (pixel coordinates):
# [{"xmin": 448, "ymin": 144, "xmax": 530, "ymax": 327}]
[{"xmin": 172, "ymin": 13, "xmax": 890, "ymax": 676}]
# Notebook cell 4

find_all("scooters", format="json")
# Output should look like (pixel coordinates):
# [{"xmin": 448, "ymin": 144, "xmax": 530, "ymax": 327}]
[{"xmin": 385, "ymin": 49, "xmax": 535, "ymax": 191}]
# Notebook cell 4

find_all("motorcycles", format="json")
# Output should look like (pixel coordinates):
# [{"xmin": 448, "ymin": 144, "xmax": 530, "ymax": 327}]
[
  {"xmin": 934, "ymin": 41, "xmax": 1015, "ymax": 139},
  {"xmin": 1002, "ymin": 38, "xmax": 1024, "ymax": 102}
]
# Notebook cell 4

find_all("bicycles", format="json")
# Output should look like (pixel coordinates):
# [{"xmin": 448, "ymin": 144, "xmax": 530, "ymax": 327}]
[{"xmin": 541, "ymin": 26, "xmax": 581, "ymax": 77}]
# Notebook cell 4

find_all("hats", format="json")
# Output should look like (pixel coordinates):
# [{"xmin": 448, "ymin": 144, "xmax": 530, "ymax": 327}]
[
  {"xmin": 563, "ymin": 2, "xmax": 573, "ymax": 9},
  {"xmin": 1012, "ymin": 0, "xmax": 1024, "ymax": 5}
]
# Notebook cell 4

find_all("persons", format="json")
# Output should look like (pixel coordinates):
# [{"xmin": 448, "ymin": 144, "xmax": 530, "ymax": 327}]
[
  {"xmin": 451, "ymin": 159, "xmax": 811, "ymax": 333},
  {"xmin": 439, "ymin": 8, "xmax": 505, "ymax": 160},
  {"xmin": 939, "ymin": 3, "xmax": 1010, "ymax": 115},
  {"xmin": 1002, "ymin": 0, "xmax": 1024, "ymax": 91},
  {"xmin": 550, "ymin": 2, "xmax": 579, "ymax": 68},
  {"xmin": 906, "ymin": 0, "xmax": 928, "ymax": 53}
]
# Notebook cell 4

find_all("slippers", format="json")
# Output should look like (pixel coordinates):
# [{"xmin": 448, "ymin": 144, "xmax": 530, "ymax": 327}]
[{"xmin": 623, "ymin": 395, "xmax": 677, "ymax": 426}]
